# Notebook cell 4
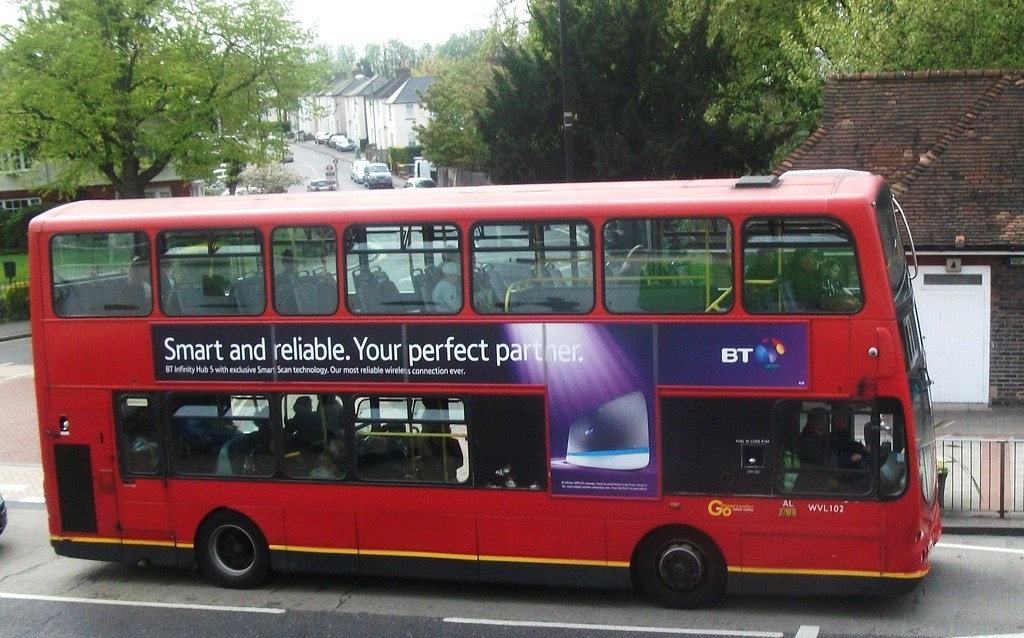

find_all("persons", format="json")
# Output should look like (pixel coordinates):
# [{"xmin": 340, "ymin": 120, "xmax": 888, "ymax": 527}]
[
  {"xmin": 791, "ymin": 407, "xmax": 872, "ymax": 494},
  {"xmin": 433, "ymin": 262, "xmax": 485, "ymax": 313},
  {"xmin": 123, "ymin": 257, "xmax": 152, "ymax": 309},
  {"xmin": 276, "ymin": 250, "xmax": 302, "ymax": 314},
  {"xmin": 746, "ymin": 244, "xmax": 854, "ymax": 314},
  {"xmin": 282, "ymin": 393, "xmax": 464, "ymax": 485},
  {"xmin": 617, "ymin": 245, "xmax": 647, "ymax": 280}
]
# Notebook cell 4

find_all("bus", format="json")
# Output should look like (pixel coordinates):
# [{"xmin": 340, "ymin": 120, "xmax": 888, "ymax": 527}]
[{"xmin": 27, "ymin": 167, "xmax": 944, "ymax": 611}]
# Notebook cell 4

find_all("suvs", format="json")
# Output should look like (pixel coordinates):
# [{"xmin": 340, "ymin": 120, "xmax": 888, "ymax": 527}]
[{"xmin": 362, "ymin": 162, "xmax": 394, "ymax": 189}]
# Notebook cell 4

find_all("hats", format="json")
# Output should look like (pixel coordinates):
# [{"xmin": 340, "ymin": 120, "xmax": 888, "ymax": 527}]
[{"xmin": 440, "ymin": 261, "xmax": 462, "ymax": 276}]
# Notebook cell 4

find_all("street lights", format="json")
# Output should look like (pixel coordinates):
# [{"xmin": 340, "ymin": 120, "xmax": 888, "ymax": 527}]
[{"xmin": 354, "ymin": 73, "xmax": 378, "ymax": 162}]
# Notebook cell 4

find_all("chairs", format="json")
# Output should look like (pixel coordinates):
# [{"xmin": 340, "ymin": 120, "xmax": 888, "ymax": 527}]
[{"xmin": 56, "ymin": 244, "xmax": 794, "ymax": 317}]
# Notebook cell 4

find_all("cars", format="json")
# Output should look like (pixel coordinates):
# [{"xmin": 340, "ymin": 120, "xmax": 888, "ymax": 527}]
[
  {"xmin": 350, "ymin": 160, "xmax": 370, "ymax": 184},
  {"xmin": 314, "ymin": 130, "xmax": 357, "ymax": 152},
  {"xmin": 283, "ymin": 146, "xmax": 294, "ymax": 163},
  {"xmin": 403, "ymin": 176, "xmax": 438, "ymax": 188},
  {"xmin": 306, "ymin": 181, "xmax": 337, "ymax": 191}
]
